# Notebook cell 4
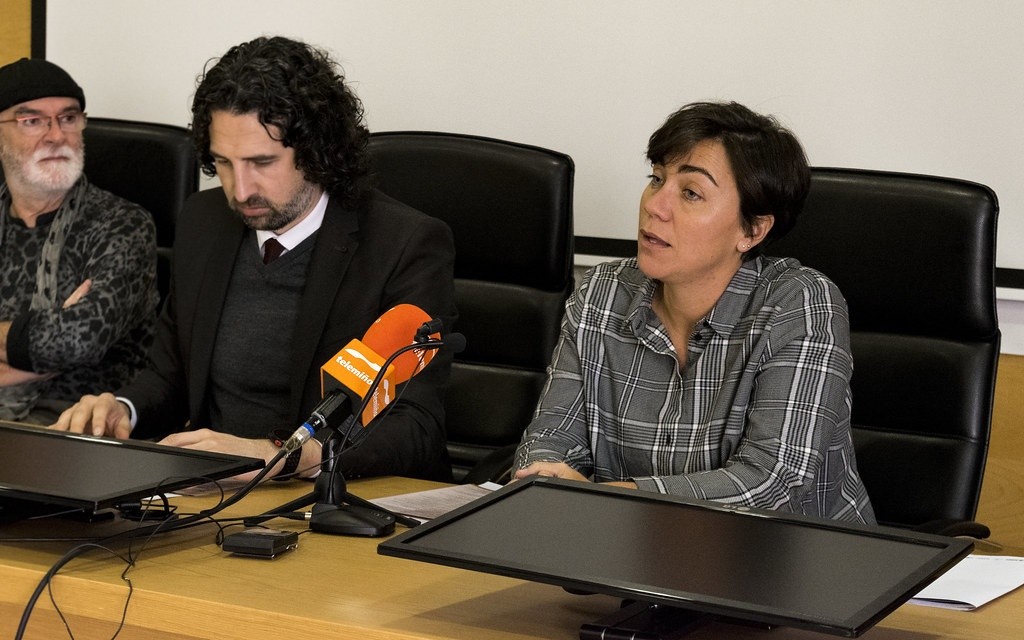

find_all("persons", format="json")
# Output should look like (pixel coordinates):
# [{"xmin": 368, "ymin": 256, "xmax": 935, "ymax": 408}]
[
  {"xmin": 0, "ymin": 57, "xmax": 161, "ymax": 427},
  {"xmin": 507, "ymin": 101, "xmax": 881, "ymax": 532},
  {"xmin": 46, "ymin": 36, "xmax": 457, "ymax": 485}
]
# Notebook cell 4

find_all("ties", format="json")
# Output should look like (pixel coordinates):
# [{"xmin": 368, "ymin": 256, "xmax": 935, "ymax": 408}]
[{"xmin": 262, "ymin": 238, "xmax": 285, "ymax": 268}]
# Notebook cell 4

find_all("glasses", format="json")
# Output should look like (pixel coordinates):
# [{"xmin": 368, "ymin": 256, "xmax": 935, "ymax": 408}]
[{"xmin": 1, "ymin": 112, "xmax": 87, "ymax": 136}]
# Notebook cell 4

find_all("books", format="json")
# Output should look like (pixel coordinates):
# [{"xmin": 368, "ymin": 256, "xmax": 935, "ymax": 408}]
[{"xmin": 907, "ymin": 554, "xmax": 1024, "ymax": 612}]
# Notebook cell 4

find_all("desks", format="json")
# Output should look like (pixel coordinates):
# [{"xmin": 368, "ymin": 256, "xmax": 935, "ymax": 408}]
[{"xmin": 0, "ymin": 477, "xmax": 1023, "ymax": 640}]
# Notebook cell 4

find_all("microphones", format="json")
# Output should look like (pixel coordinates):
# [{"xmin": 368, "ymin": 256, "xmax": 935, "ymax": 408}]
[
  {"xmin": 282, "ymin": 304, "xmax": 442, "ymax": 454},
  {"xmin": 308, "ymin": 332, "xmax": 466, "ymax": 538},
  {"xmin": 414, "ymin": 319, "xmax": 444, "ymax": 345}
]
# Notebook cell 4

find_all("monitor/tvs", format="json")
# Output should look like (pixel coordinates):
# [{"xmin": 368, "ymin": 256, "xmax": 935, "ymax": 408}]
[
  {"xmin": 0, "ymin": 420, "xmax": 266, "ymax": 522},
  {"xmin": 377, "ymin": 472, "xmax": 976, "ymax": 640}
]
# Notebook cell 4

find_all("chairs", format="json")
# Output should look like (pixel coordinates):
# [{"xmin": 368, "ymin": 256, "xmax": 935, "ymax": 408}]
[
  {"xmin": 768, "ymin": 166, "xmax": 1001, "ymax": 538},
  {"xmin": 364, "ymin": 131, "xmax": 575, "ymax": 484},
  {"xmin": 81, "ymin": 118, "xmax": 199, "ymax": 283}
]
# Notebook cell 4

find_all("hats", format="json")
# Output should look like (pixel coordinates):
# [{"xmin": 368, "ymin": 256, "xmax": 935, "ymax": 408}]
[{"xmin": 0, "ymin": 57, "xmax": 86, "ymax": 113}]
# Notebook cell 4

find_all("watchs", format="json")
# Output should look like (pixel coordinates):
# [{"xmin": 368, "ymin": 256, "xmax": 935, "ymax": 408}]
[{"xmin": 260, "ymin": 427, "xmax": 302, "ymax": 481}]
